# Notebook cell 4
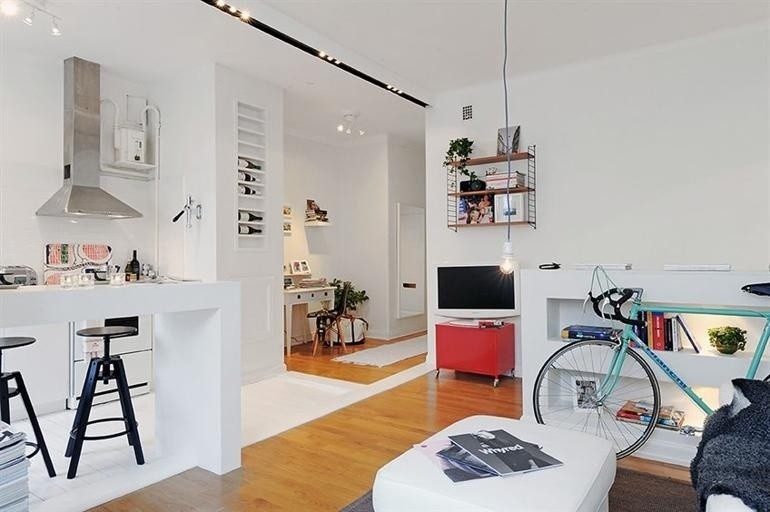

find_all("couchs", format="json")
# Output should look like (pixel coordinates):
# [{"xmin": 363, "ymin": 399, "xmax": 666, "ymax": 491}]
[{"xmin": 697, "ymin": 376, "xmax": 769, "ymax": 512}]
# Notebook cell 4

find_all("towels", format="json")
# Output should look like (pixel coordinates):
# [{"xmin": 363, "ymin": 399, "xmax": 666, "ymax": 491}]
[{"xmin": 81, "ymin": 317, "xmax": 104, "ymax": 362}]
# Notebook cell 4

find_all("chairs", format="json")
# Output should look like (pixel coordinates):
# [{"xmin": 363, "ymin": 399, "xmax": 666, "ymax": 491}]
[{"xmin": 306, "ymin": 282, "xmax": 352, "ymax": 355}]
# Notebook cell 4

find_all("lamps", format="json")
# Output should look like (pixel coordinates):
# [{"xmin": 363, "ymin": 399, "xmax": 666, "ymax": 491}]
[
  {"xmin": 494, "ymin": 0, "xmax": 522, "ymax": 276},
  {"xmin": 335, "ymin": 112, "xmax": 367, "ymax": 138}
]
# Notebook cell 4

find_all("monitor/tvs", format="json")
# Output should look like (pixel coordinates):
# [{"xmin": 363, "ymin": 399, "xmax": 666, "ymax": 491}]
[{"xmin": 435, "ymin": 265, "xmax": 520, "ymax": 326}]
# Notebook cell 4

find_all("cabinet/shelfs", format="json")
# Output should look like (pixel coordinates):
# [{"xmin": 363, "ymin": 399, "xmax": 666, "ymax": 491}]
[
  {"xmin": 445, "ymin": 143, "xmax": 535, "ymax": 233},
  {"xmin": 436, "ymin": 320, "xmax": 516, "ymax": 388},
  {"xmin": 235, "ymin": 97, "xmax": 268, "ymax": 251},
  {"xmin": 519, "ymin": 266, "xmax": 769, "ymax": 472}
]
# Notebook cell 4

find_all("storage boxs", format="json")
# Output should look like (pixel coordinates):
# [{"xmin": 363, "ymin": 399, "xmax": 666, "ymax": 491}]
[{"xmin": 484, "ymin": 170, "xmax": 525, "ymax": 189}]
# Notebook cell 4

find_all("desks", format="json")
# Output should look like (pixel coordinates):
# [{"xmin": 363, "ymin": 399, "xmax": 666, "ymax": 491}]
[
  {"xmin": 0, "ymin": 275, "xmax": 247, "ymax": 482},
  {"xmin": 284, "ymin": 285, "xmax": 338, "ymax": 360}
]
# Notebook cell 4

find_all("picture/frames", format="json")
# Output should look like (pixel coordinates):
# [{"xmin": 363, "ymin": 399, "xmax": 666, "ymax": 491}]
[
  {"xmin": 282, "ymin": 220, "xmax": 294, "ymax": 234},
  {"xmin": 291, "ymin": 259, "xmax": 302, "ymax": 275},
  {"xmin": 569, "ymin": 375, "xmax": 605, "ymax": 416},
  {"xmin": 282, "ymin": 202, "xmax": 294, "ymax": 219},
  {"xmin": 284, "ymin": 276, "xmax": 294, "ymax": 287},
  {"xmin": 300, "ymin": 260, "xmax": 311, "ymax": 274}
]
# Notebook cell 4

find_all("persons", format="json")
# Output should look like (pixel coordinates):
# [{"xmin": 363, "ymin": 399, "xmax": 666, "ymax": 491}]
[{"xmin": 466, "ymin": 195, "xmax": 491, "ymax": 224}]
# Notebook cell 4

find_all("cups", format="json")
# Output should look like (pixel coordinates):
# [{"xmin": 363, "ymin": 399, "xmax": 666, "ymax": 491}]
[
  {"xmin": 130, "ymin": 274, "xmax": 137, "ymax": 282},
  {"xmin": 148, "ymin": 270, "xmax": 155, "ymax": 279},
  {"xmin": 142, "ymin": 264, "xmax": 150, "ymax": 276},
  {"xmin": 60, "ymin": 273, "xmax": 126, "ymax": 288}
]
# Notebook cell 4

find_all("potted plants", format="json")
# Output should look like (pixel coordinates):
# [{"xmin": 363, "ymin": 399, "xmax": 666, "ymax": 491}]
[
  {"xmin": 443, "ymin": 133, "xmax": 477, "ymax": 179},
  {"xmin": 708, "ymin": 324, "xmax": 748, "ymax": 355}
]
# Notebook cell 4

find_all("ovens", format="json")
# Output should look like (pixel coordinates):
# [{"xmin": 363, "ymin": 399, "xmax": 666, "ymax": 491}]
[{"xmin": 83, "ymin": 313, "xmax": 153, "ymax": 363}]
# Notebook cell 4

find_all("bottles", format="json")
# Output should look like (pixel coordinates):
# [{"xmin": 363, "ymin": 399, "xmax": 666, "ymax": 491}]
[
  {"xmin": 239, "ymin": 212, "xmax": 262, "ymax": 222},
  {"xmin": 238, "ymin": 172, "xmax": 262, "ymax": 183},
  {"xmin": 239, "ymin": 185, "xmax": 262, "ymax": 195},
  {"xmin": 238, "ymin": 159, "xmax": 261, "ymax": 170},
  {"xmin": 131, "ymin": 250, "xmax": 140, "ymax": 279},
  {"xmin": 239, "ymin": 226, "xmax": 262, "ymax": 235},
  {"xmin": 125, "ymin": 256, "xmax": 132, "ymax": 280}
]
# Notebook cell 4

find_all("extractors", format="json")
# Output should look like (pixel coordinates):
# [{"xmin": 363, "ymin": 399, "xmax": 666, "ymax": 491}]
[{"xmin": 34, "ymin": 58, "xmax": 144, "ymax": 219}]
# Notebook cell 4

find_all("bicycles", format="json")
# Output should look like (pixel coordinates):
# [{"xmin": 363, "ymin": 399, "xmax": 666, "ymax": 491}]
[{"xmin": 530, "ymin": 281, "xmax": 769, "ymax": 462}]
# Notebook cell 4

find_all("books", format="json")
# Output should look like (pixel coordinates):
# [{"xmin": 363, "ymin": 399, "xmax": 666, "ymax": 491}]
[{"xmin": 561, "ymin": 309, "xmax": 685, "ymax": 431}]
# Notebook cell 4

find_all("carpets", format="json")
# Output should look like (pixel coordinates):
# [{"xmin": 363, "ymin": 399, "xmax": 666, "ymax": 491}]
[
  {"xmin": 334, "ymin": 331, "xmax": 427, "ymax": 368},
  {"xmin": 337, "ymin": 462, "xmax": 701, "ymax": 512}
]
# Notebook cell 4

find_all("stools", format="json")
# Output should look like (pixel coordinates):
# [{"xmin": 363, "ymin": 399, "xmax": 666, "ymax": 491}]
[
  {"xmin": 63, "ymin": 323, "xmax": 145, "ymax": 482},
  {"xmin": 0, "ymin": 334, "xmax": 56, "ymax": 478},
  {"xmin": 372, "ymin": 411, "xmax": 618, "ymax": 512}
]
